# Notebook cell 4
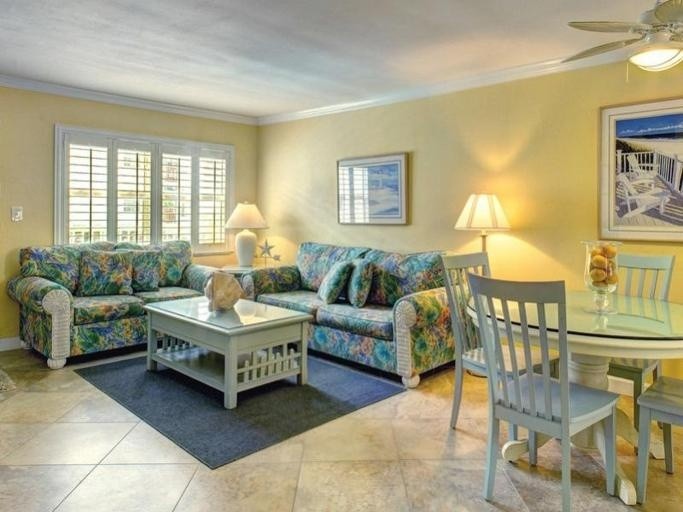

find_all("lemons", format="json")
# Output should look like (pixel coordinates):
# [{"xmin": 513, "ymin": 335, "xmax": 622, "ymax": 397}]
[{"xmin": 589, "ymin": 244, "xmax": 617, "ymax": 288}]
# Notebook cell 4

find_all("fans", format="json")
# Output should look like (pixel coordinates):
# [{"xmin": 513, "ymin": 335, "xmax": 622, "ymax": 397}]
[{"xmin": 560, "ymin": 0, "xmax": 683, "ymax": 62}]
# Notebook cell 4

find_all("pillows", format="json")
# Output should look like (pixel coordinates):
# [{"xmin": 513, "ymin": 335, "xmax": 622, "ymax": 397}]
[
  {"xmin": 345, "ymin": 257, "xmax": 374, "ymax": 308},
  {"xmin": 80, "ymin": 250, "xmax": 134, "ymax": 295},
  {"xmin": 317, "ymin": 260, "xmax": 352, "ymax": 305},
  {"xmin": 20, "ymin": 244, "xmax": 81, "ymax": 297},
  {"xmin": 144, "ymin": 240, "xmax": 193, "ymax": 286},
  {"xmin": 114, "ymin": 249, "xmax": 162, "ymax": 292}
]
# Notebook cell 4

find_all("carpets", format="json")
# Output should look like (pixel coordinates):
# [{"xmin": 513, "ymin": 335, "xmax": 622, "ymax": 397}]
[{"xmin": 74, "ymin": 345, "xmax": 406, "ymax": 470}]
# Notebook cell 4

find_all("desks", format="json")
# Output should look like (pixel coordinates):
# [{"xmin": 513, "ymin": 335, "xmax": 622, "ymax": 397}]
[{"xmin": 466, "ymin": 291, "xmax": 683, "ymax": 506}]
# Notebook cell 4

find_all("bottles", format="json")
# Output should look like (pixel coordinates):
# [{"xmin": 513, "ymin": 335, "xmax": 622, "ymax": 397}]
[{"xmin": 204, "ymin": 270, "xmax": 242, "ymax": 312}]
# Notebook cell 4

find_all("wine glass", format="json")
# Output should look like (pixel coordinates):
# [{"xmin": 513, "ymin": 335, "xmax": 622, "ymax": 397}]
[{"xmin": 580, "ymin": 240, "xmax": 621, "ymax": 314}]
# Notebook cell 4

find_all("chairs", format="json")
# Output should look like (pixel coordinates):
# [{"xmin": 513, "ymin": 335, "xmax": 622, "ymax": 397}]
[
  {"xmin": 437, "ymin": 253, "xmax": 559, "ymax": 463},
  {"xmin": 606, "ymin": 294, "xmax": 672, "ymax": 336},
  {"xmin": 468, "ymin": 271, "xmax": 621, "ymax": 512},
  {"xmin": 638, "ymin": 376, "xmax": 683, "ymax": 504},
  {"xmin": 615, "ymin": 154, "xmax": 672, "ymax": 225},
  {"xmin": 607, "ymin": 253, "xmax": 675, "ymax": 456}
]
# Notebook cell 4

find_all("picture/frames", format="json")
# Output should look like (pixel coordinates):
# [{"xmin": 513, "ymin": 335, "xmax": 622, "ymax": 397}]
[
  {"xmin": 597, "ymin": 95, "xmax": 683, "ymax": 244},
  {"xmin": 337, "ymin": 151, "xmax": 409, "ymax": 226}
]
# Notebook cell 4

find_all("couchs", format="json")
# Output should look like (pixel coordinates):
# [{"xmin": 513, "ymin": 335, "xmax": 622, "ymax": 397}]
[
  {"xmin": 6, "ymin": 239, "xmax": 224, "ymax": 370},
  {"xmin": 242, "ymin": 242, "xmax": 482, "ymax": 389}
]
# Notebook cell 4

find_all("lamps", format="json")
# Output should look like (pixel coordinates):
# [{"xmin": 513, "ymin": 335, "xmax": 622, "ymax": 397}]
[
  {"xmin": 454, "ymin": 192, "xmax": 512, "ymax": 276},
  {"xmin": 224, "ymin": 201, "xmax": 269, "ymax": 267},
  {"xmin": 627, "ymin": 32, "xmax": 683, "ymax": 71}
]
